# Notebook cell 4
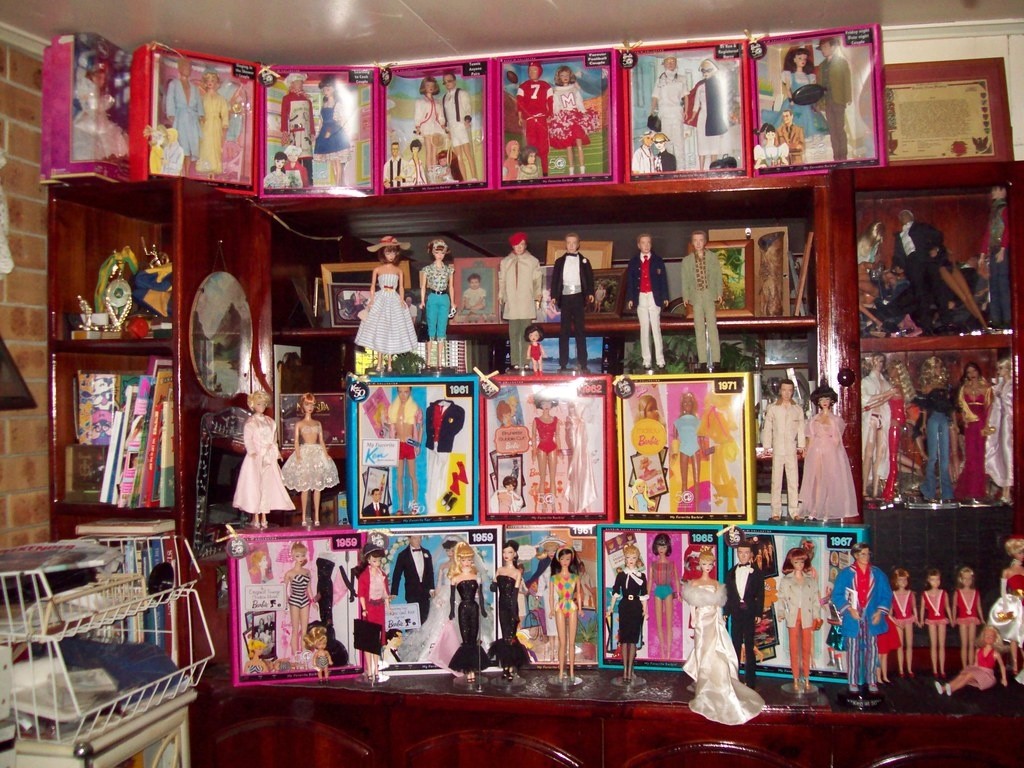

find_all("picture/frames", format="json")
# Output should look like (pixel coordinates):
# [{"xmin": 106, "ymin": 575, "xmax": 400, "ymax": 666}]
[
  {"xmin": 548, "ymin": 238, "xmax": 612, "ymax": 270},
  {"xmin": 450, "ymin": 258, "xmax": 501, "ymax": 326},
  {"xmin": 584, "ymin": 266, "xmax": 628, "ymax": 321},
  {"xmin": 318, "ymin": 260, "xmax": 412, "ymax": 311},
  {"xmin": 684, "ymin": 238, "xmax": 754, "ymax": 321},
  {"xmin": 327, "ymin": 282, "xmax": 382, "ymax": 329}
]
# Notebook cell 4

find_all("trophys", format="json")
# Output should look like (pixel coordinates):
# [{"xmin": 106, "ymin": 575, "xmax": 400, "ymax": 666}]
[{"xmin": 71, "ymin": 236, "xmax": 172, "ymax": 339}]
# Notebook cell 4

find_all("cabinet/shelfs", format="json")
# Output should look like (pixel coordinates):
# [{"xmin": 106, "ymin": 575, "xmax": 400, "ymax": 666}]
[{"xmin": 36, "ymin": 169, "xmax": 1024, "ymax": 768}]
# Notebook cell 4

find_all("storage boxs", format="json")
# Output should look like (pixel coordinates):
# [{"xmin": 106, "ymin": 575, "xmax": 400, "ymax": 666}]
[{"xmin": 34, "ymin": 22, "xmax": 882, "ymax": 694}]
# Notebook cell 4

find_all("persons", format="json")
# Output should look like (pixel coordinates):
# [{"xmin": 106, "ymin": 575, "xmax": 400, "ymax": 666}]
[
  {"xmin": 75, "ymin": 47, "xmax": 350, "ymax": 184},
  {"xmin": 672, "ymin": 393, "xmax": 707, "ymax": 512},
  {"xmin": 354, "ymin": 232, "xmax": 548, "ymax": 376},
  {"xmin": 761, "ymin": 352, "xmax": 1013, "ymax": 524},
  {"xmin": 830, "ymin": 534, "xmax": 1024, "ymax": 696},
  {"xmin": 549, "ymin": 230, "xmax": 724, "ymax": 373},
  {"xmin": 857, "ymin": 186, "xmax": 1010, "ymax": 337},
  {"xmin": 593, "ymin": 282, "xmax": 606, "ymax": 312},
  {"xmin": 463, "ymin": 272, "xmax": 486, "ymax": 310},
  {"xmin": 549, "ymin": 534, "xmax": 823, "ymax": 726},
  {"xmin": 284, "ymin": 534, "xmax": 531, "ymax": 685},
  {"xmin": 232, "ymin": 390, "xmax": 340, "ymax": 527},
  {"xmin": 389, "ymin": 387, "xmax": 422, "ymax": 516},
  {"xmin": 531, "ymin": 392, "xmax": 564, "ymax": 513},
  {"xmin": 414, "ymin": 38, "xmax": 851, "ymax": 185}
]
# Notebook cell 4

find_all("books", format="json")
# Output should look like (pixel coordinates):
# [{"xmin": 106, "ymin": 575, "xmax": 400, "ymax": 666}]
[
  {"xmin": 64, "ymin": 356, "xmax": 175, "ymax": 508},
  {"xmin": 76, "ymin": 519, "xmax": 178, "ymax": 666},
  {"xmin": 13, "ymin": 668, "xmax": 123, "ymax": 723}
]
